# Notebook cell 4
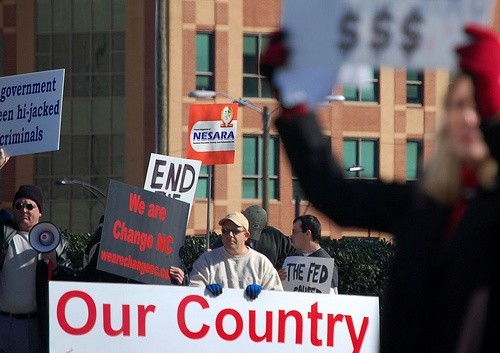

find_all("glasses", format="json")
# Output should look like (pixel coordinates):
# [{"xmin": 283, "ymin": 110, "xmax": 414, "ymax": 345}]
[
  {"xmin": 292, "ymin": 229, "xmax": 303, "ymax": 235},
  {"xmin": 15, "ymin": 202, "xmax": 36, "ymax": 210},
  {"xmin": 222, "ymin": 229, "xmax": 246, "ymax": 236}
]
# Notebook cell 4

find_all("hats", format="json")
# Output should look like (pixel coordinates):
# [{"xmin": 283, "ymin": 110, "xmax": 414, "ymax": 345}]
[
  {"xmin": 242, "ymin": 205, "xmax": 268, "ymax": 240},
  {"xmin": 218, "ymin": 212, "xmax": 249, "ymax": 231},
  {"xmin": 12, "ymin": 185, "xmax": 42, "ymax": 213}
]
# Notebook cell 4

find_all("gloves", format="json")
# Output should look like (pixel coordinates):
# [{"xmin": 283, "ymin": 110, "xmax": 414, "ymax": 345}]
[
  {"xmin": 259, "ymin": 26, "xmax": 314, "ymax": 115},
  {"xmin": 206, "ymin": 284, "xmax": 223, "ymax": 296},
  {"xmin": 454, "ymin": 24, "xmax": 500, "ymax": 116},
  {"xmin": 246, "ymin": 285, "xmax": 262, "ymax": 300}
]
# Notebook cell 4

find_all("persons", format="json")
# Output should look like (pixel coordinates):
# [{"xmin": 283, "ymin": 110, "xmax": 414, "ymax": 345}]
[
  {"xmin": 83, "ymin": 215, "xmax": 110, "ymax": 282},
  {"xmin": 170, "ymin": 254, "xmax": 189, "ymax": 286},
  {"xmin": 187, "ymin": 211, "xmax": 285, "ymax": 300},
  {"xmin": 277, "ymin": 214, "xmax": 338, "ymax": 295},
  {"xmin": 0, "ymin": 148, "xmax": 80, "ymax": 353},
  {"xmin": 257, "ymin": 23, "xmax": 500, "ymax": 353},
  {"xmin": 212, "ymin": 204, "xmax": 302, "ymax": 267}
]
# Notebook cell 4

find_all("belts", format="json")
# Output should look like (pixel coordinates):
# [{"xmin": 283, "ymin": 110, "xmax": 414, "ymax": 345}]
[{"xmin": 0, "ymin": 311, "xmax": 38, "ymax": 320}]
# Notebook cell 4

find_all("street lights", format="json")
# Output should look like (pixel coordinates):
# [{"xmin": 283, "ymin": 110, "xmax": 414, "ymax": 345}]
[
  {"xmin": 187, "ymin": 89, "xmax": 346, "ymax": 220},
  {"xmin": 56, "ymin": 178, "xmax": 108, "ymax": 208}
]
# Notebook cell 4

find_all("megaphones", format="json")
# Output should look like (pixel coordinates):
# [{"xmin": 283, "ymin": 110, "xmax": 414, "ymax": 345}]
[{"xmin": 28, "ymin": 221, "xmax": 61, "ymax": 253}]
